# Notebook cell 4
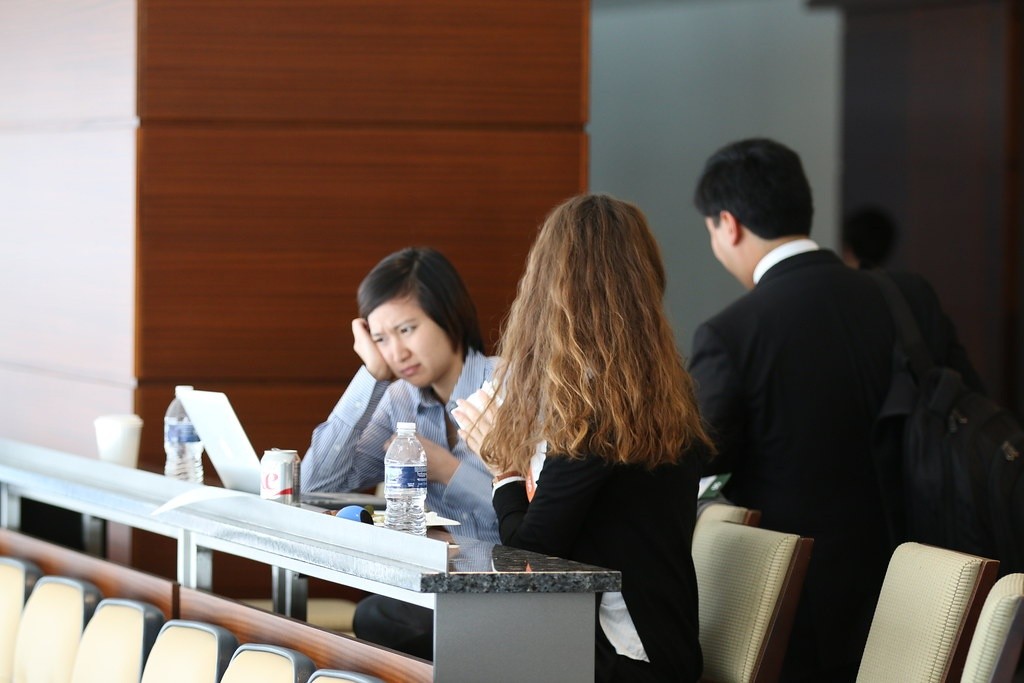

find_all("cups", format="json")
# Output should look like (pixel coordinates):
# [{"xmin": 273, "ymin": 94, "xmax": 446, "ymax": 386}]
[{"xmin": 94, "ymin": 414, "xmax": 143, "ymax": 469}]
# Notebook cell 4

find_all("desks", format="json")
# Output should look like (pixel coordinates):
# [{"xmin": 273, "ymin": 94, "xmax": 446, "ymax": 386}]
[{"xmin": 0, "ymin": 437, "xmax": 623, "ymax": 683}]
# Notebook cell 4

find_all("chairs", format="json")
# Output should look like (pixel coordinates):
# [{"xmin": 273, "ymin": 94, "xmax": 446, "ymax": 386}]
[
  {"xmin": 693, "ymin": 501, "xmax": 1024, "ymax": 683},
  {"xmin": 0, "ymin": 555, "xmax": 386, "ymax": 683}
]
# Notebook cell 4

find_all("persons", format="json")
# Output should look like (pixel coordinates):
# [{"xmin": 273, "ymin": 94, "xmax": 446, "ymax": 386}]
[
  {"xmin": 683, "ymin": 138, "xmax": 985, "ymax": 683},
  {"xmin": 295, "ymin": 246, "xmax": 549, "ymax": 660},
  {"xmin": 839, "ymin": 203, "xmax": 980, "ymax": 393},
  {"xmin": 485, "ymin": 193, "xmax": 722, "ymax": 683}
]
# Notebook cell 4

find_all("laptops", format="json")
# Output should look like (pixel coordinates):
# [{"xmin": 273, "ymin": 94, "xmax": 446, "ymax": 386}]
[{"xmin": 176, "ymin": 390, "xmax": 387, "ymax": 511}]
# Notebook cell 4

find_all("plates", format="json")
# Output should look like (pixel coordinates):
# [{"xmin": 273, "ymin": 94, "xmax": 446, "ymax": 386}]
[{"xmin": 372, "ymin": 510, "xmax": 461, "ymax": 527}]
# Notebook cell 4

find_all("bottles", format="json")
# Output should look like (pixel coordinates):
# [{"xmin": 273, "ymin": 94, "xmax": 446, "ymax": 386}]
[
  {"xmin": 384, "ymin": 422, "xmax": 428, "ymax": 536},
  {"xmin": 163, "ymin": 385, "xmax": 204, "ymax": 483}
]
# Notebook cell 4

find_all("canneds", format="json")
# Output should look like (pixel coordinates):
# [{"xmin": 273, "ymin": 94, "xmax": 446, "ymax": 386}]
[{"xmin": 259, "ymin": 447, "xmax": 300, "ymax": 508}]
